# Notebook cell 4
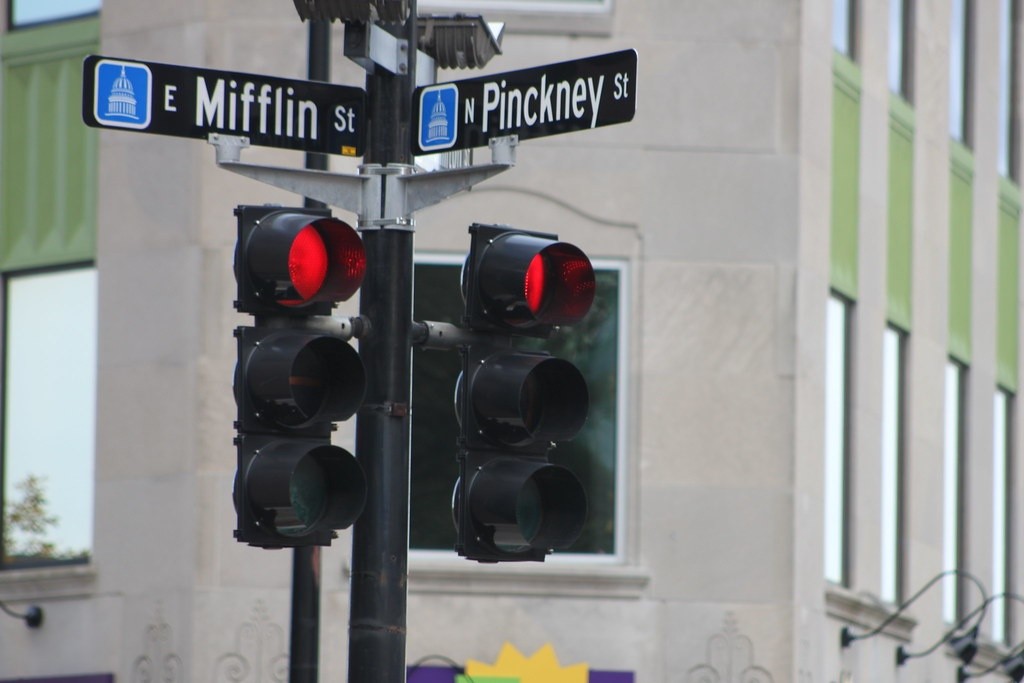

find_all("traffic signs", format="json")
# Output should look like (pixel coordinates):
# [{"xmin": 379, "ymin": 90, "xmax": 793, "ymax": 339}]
[
  {"xmin": 82, "ymin": 54, "xmax": 367, "ymax": 157},
  {"xmin": 411, "ymin": 48, "xmax": 638, "ymax": 156}
]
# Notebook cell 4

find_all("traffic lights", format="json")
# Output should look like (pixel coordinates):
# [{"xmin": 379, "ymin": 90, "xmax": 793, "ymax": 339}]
[
  {"xmin": 232, "ymin": 203, "xmax": 368, "ymax": 549},
  {"xmin": 451, "ymin": 223, "xmax": 595, "ymax": 563}
]
